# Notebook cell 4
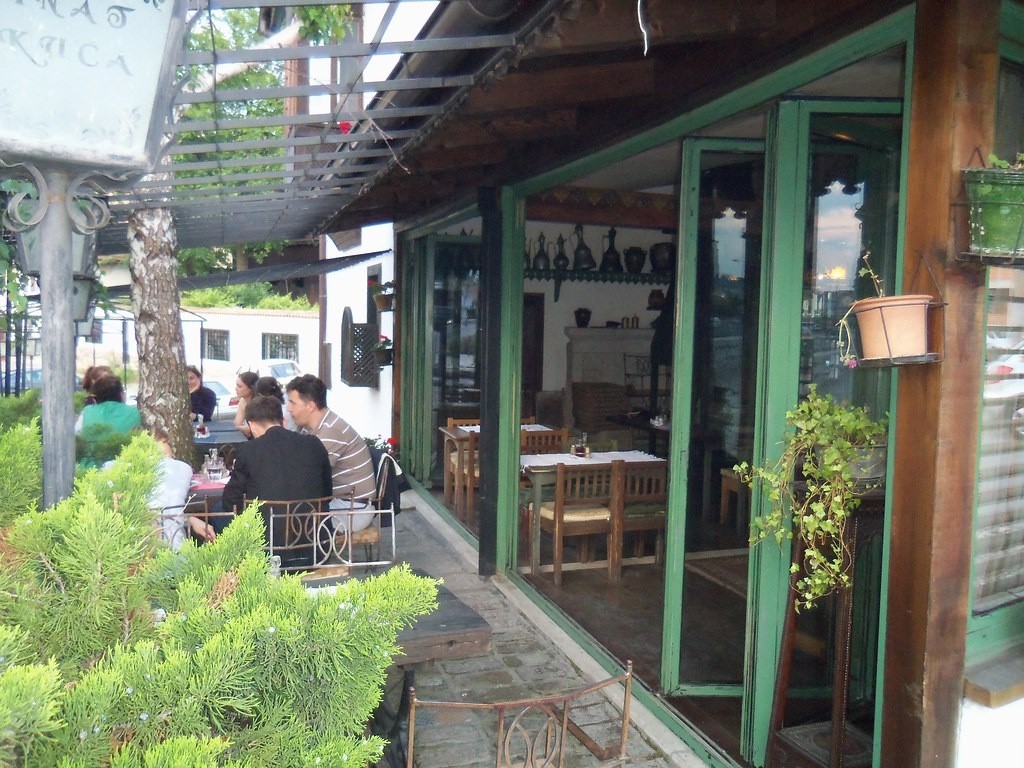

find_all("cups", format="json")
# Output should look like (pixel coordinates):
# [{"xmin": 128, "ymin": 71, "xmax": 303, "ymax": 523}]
[{"xmin": 205, "ymin": 456, "xmax": 224, "ymax": 481}]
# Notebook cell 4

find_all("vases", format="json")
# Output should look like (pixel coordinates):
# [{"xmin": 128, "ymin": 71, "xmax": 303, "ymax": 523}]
[
  {"xmin": 372, "ymin": 349, "xmax": 393, "ymax": 363},
  {"xmin": 373, "ymin": 294, "xmax": 394, "ymax": 313}
]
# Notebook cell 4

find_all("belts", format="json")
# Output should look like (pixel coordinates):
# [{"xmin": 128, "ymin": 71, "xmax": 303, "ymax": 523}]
[{"xmin": 341, "ymin": 497, "xmax": 376, "ymax": 505}]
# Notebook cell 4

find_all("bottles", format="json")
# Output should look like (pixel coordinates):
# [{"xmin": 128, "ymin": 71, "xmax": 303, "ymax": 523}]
[
  {"xmin": 571, "ymin": 432, "xmax": 590, "ymax": 458},
  {"xmin": 201, "ymin": 448, "xmax": 218, "ymax": 476},
  {"xmin": 194, "ymin": 414, "xmax": 210, "ymax": 438},
  {"xmin": 652, "ymin": 414, "xmax": 667, "ymax": 427}
]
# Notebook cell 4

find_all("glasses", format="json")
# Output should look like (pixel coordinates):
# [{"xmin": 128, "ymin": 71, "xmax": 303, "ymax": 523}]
[
  {"xmin": 188, "ymin": 365, "xmax": 197, "ymax": 371},
  {"xmin": 120, "ymin": 387, "xmax": 126, "ymax": 396}
]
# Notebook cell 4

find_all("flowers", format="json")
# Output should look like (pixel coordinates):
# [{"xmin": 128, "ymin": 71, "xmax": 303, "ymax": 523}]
[
  {"xmin": 833, "ymin": 302, "xmax": 857, "ymax": 369},
  {"xmin": 371, "ymin": 335, "xmax": 394, "ymax": 353},
  {"xmin": 368, "ymin": 280, "xmax": 396, "ymax": 295}
]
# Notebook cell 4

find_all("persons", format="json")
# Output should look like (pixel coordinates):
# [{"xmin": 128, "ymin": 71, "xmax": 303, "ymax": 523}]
[
  {"xmin": 285, "ymin": 374, "xmax": 376, "ymax": 536},
  {"xmin": 187, "ymin": 366, "xmax": 216, "ymax": 423},
  {"xmin": 233, "ymin": 372, "xmax": 298, "ymax": 441},
  {"xmin": 75, "ymin": 366, "xmax": 142, "ymax": 470},
  {"xmin": 97, "ymin": 423, "xmax": 215, "ymax": 588},
  {"xmin": 223, "ymin": 396, "xmax": 334, "ymax": 578}
]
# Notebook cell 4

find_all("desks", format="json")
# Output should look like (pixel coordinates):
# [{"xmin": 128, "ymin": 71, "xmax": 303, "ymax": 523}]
[
  {"xmin": 438, "ymin": 423, "xmax": 577, "ymax": 522},
  {"xmin": 193, "ymin": 420, "xmax": 250, "ymax": 446},
  {"xmin": 605, "ymin": 413, "xmax": 755, "ymax": 520},
  {"xmin": 301, "ymin": 568, "xmax": 492, "ymax": 768},
  {"xmin": 520, "ymin": 448, "xmax": 666, "ymax": 576},
  {"xmin": 184, "ymin": 471, "xmax": 231, "ymax": 513}
]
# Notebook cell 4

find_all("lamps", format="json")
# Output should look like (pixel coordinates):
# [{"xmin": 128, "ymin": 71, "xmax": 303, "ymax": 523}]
[{"xmin": 0, "ymin": 0, "xmax": 187, "ymax": 338}]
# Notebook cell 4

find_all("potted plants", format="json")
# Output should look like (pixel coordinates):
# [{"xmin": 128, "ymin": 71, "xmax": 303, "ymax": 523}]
[
  {"xmin": 733, "ymin": 382, "xmax": 888, "ymax": 614},
  {"xmin": 961, "ymin": 151, "xmax": 1024, "ymax": 258},
  {"xmin": 852, "ymin": 249, "xmax": 933, "ymax": 359}
]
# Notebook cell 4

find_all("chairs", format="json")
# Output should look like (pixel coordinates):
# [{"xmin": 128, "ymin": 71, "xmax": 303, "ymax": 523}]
[
  {"xmin": 720, "ymin": 467, "xmax": 751, "ymax": 525},
  {"xmin": 448, "ymin": 417, "xmax": 667, "ymax": 585},
  {"xmin": 147, "ymin": 444, "xmax": 401, "ymax": 585},
  {"xmin": 403, "ymin": 658, "xmax": 634, "ymax": 768}
]
